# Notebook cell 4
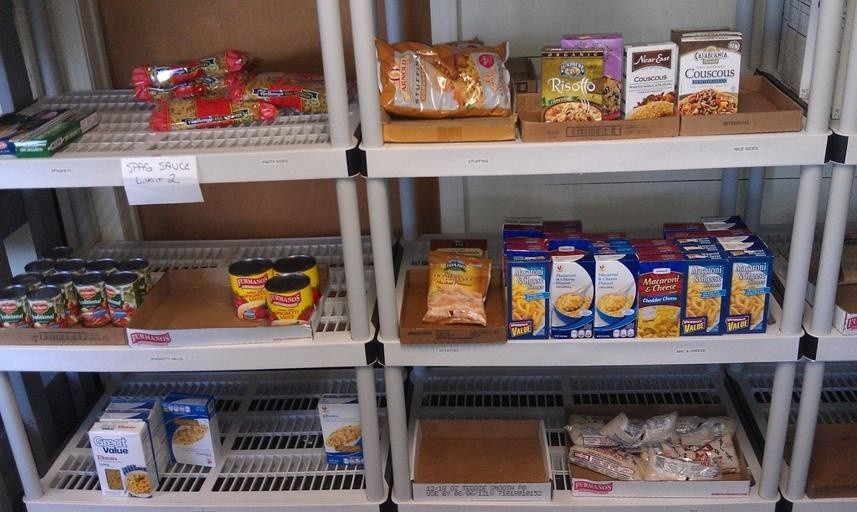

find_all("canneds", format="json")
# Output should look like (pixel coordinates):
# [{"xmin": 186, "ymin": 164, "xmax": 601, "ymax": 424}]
[
  {"xmin": 85, "ymin": 258, "xmax": 119, "ymax": 276},
  {"xmin": 0, "ymin": 284, "xmax": 27, "ymax": 329},
  {"xmin": 227, "ymin": 257, "xmax": 273, "ymax": 323},
  {"xmin": 104, "ymin": 273, "xmax": 142, "ymax": 328},
  {"xmin": 26, "ymin": 285, "xmax": 67, "ymax": 329},
  {"xmin": 24, "ymin": 258, "xmax": 55, "ymax": 276},
  {"xmin": 43, "ymin": 270, "xmax": 82, "ymax": 326},
  {"xmin": 272, "ymin": 255, "xmax": 321, "ymax": 304},
  {"xmin": 116, "ymin": 258, "xmax": 153, "ymax": 302},
  {"xmin": 54, "ymin": 257, "xmax": 86, "ymax": 273},
  {"xmin": 42, "ymin": 244, "xmax": 73, "ymax": 263},
  {"xmin": 12, "ymin": 273, "xmax": 44, "ymax": 290},
  {"xmin": 72, "ymin": 273, "xmax": 111, "ymax": 328},
  {"xmin": 264, "ymin": 273, "xmax": 315, "ymax": 325}
]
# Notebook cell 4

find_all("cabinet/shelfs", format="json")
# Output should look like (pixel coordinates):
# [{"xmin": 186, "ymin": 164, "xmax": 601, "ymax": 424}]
[
  {"xmin": 727, "ymin": 0, "xmax": 857, "ymax": 512},
  {"xmin": 0, "ymin": 0, "xmax": 406, "ymax": 512},
  {"xmin": 349, "ymin": 1, "xmax": 846, "ymax": 512}
]
